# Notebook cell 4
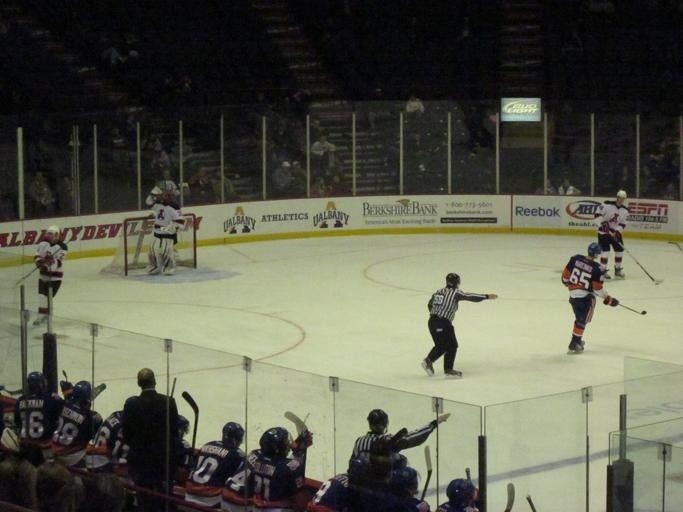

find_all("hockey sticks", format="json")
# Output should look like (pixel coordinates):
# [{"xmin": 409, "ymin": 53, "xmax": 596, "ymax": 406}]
[
  {"xmin": 595, "ymin": 293, "xmax": 647, "ymax": 315},
  {"xmin": 90, "ymin": 383, "xmax": 106, "ymax": 401},
  {"xmin": 2, "ymin": 386, "xmax": 23, "ymax": 395},
  {"xmin": 607, "ymin": 228, "xmax": 664, "ymax": 284},
  {"xmin": 62, "ymin": 370, "xmax": 67, "ymax": 382},
  {"xmin": 181, "ymin": 391, "xmax": 198, "ymax": 453},
  {"xmin": 505, "ymin": 483, "xmax": 515, "ymax": 512},
  {"xmin": 283, "ymin": 411, "xmax": 308, "ymax": 478},
  {"xmin": 419, "ymin": 446, "xmax": 432, "ymax": 502},
  {"xmin": 15, "ymin": 225, "xmax": 83, "ymax": 288}
]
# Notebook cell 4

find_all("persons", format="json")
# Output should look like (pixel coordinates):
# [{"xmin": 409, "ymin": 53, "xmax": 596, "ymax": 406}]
[
  {"xmin": 424, "ymin": 273, "xmax": 498, "ymax": 376},
  {"xmin": 593, "ymin": 189, "xmax": 629, "ymax": 280},
  {"xmin": 31, "ymin": 225, "xmax": 68, "ymax": 326},
  {"xmin": 145, "ymin": 192, "xmax": 187, "ymax": 275},
  {"xmin": 561, "ymin": 241, "xmax": 618, "ymax": 351}
]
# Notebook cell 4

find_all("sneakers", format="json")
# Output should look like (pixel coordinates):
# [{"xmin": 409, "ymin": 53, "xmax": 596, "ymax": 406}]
[
  {"xmin": 34, "ymin": 316, "xmax": 48, "ymax": 325},
  {"xmin": 423, "ymin": 357, "xmax": 435, "ymax": 373},
  {"xmin": 614, "ymin": 268, "xmax": 625, "ymax": 277},
  {"xmin": 445, "ymin": 369, "xmax": 462, "ymax": 375},
  {"xmin": 577, "ymin": 339, "xmax": 585, "ymax": 344},
  {"xmin": 568, "ymin": 342, "xmax": 583, "ymax": 350},
  {"xmin": 603, "ymin": 268, "xmax": 611, "ymax": 279}
]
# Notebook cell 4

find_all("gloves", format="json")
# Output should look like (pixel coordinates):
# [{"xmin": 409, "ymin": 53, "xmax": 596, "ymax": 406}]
[
  {"xmin": 291, "ymin": 431, "xmax": 314, "ymax": 452},
  {"xmin": 601, "ymin": 222, "xmax": 610, "ymax": 232},
  {"xmin": 602, "ymin": 296, "xmax": 618, "ymax": 306},
  {"xmin": 613, "ymin": 231, "xmax": 622, "ymax": 242}
]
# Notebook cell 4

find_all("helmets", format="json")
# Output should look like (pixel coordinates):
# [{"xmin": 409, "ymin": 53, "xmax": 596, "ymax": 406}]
[
  {"xmin": 25, "ymin": 372, "xmax": 48, "ymax": 393},
  {"xmin": 72, "ymin": 381, "xmax": 91, "ymax": 404},
  {"xmin": 259, "ymin": 426, "xmax": 293, "ymax": 461},
  {"xmin": 348, "ymin": 457, "xmax": 371, "ymax": 478},
  {"xmin": 446, "ymin": 273, "xmax": 460, "ymax": 288},
  {"xmin": 447, "ymin": 479, "xmax": 474, "ymax": 507},
  {"xmin": 368, "ymin": 409, "xmax": 389, "ymax": 434},
  {"xmin": 47, "ymin": 226, "xmax": 60, "ymax": 243},
  {"xmin": 223, "ymin": 422, "xmax": 243, "ymax": 445},
  {"xmin": 587, "ymin": 242, "xmax": 602, "ymax": 258},
  {"xmin": 178, "ymin": 415, "xmax": 189, "ymax": 436},
  {"xmin": 390, "ymin": 467, "xmax": 421, "ymax": 493},
  {"xmin": 617, "ymin": 190, "xmax": 627, "ymax": 203}
]
